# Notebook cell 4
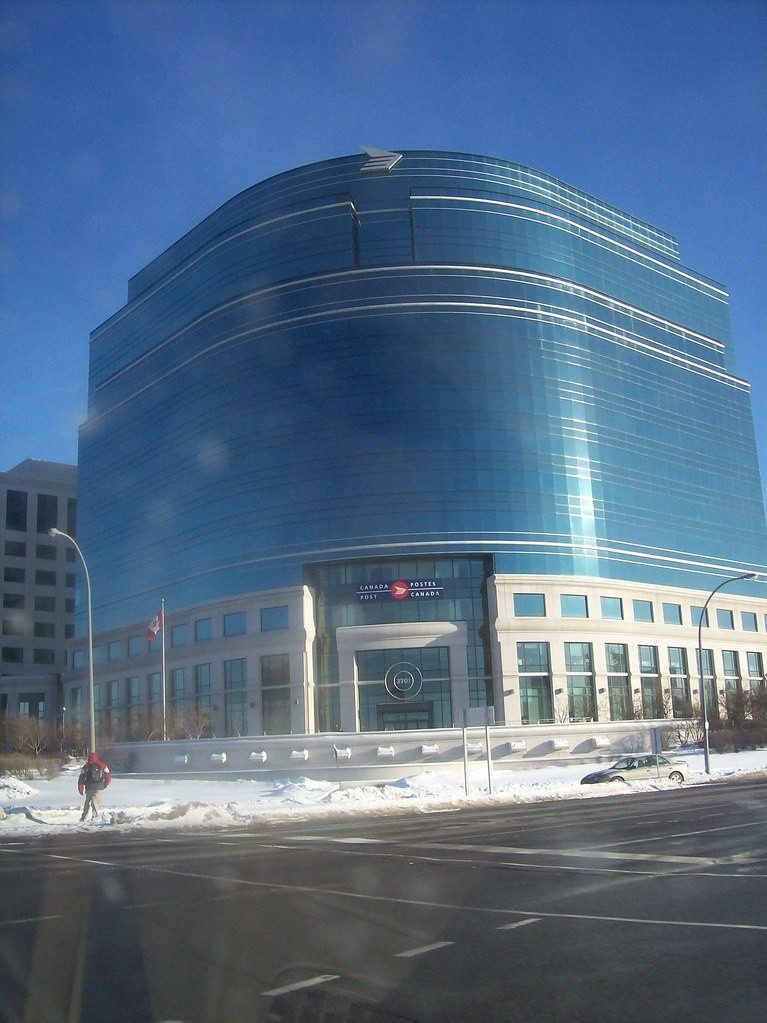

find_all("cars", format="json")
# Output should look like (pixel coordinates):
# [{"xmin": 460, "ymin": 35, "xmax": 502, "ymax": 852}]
[{"xmin": 581, "ymin": 754, "xmax": 690, "ymax": 786}]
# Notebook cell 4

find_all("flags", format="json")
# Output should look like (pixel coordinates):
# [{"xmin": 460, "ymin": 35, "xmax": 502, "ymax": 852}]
[{"xmin": 147, "ymin": 607, "xmax": 162, "ymax": 641}]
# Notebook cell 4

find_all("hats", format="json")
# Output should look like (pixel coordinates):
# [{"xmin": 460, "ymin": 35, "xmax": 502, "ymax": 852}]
[{"xmin": 89, "ymin": 753, "xmax": 96, "ymax": 761}]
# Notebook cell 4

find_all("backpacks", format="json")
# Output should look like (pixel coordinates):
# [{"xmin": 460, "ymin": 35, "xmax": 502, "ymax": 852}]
[{"xmin": 87, "ymin": 762, "xmax": 105, "ymax": 783}]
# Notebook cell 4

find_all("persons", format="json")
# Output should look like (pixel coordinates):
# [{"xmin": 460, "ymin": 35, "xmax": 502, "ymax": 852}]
[{"xmin": 78, "ymin": 753, "xmax": 112, "ymax": 822}]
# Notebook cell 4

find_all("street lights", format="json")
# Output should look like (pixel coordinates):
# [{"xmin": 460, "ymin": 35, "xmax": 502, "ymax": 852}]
[
  {"xmin": 699, "ymin": 572, "xmax": 758, "ymax": 774},
  {"xmin": 49, "ymin": 527, "xmax": 96, "ymax": 756}
]
